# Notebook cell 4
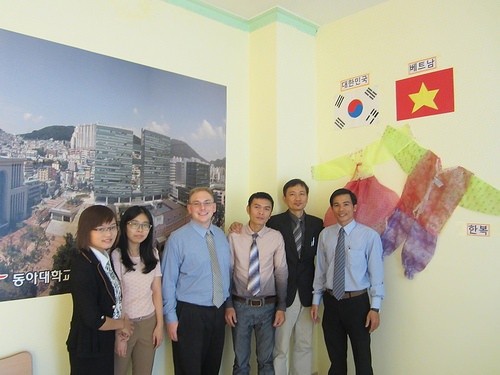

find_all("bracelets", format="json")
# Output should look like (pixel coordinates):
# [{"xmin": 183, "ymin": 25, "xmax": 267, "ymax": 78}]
[{"xmin": 370, "ymin": 308, "xmax": 379, "ymax": 313}]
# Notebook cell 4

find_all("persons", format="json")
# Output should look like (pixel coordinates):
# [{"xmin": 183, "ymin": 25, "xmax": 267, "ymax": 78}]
[
  {"xmin": 109, "ymin": 206, "xmax": 163, "ymax": 375},
  {"xmin": 226, "ymin": 192, "xmax": 289, "ymax": 375},
  {"xmin": 66, "ymin": 205, "xmax": 134, "ymax": 375},
  {"xmin": 310, "ymin": 188, "xmax": 384, "ymax": 375},
  {"xmin": 162, "ymin": 187, "xmax": 232, "ymax": 375},
  {"xmin": 229, "ymin": 179, "xmax": 325, "ymax": 375}
]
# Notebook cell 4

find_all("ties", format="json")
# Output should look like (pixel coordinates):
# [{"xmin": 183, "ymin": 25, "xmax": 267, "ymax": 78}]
[
  {"xmin": 293, "ymin": 219, "xmax": 302, "ymax": 259},
  {"xmin": 333, "ymin": 228, "xmax": 346, "ymax": 301},
  {"xmin": 204, "ymin": 230, "xmax": 225, "ymax": 309},
  {"xmin": 246, "ymin": 233, "xmax": 260, "ymax": 296}
]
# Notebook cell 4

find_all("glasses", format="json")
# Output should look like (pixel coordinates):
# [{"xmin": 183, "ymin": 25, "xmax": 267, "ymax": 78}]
[
  {"xmin": 127, "ymin": 222, "xmax": 152, "ymax": 231},
  {"xmin": 188, "ymin": 199, "xmax": 214, "ymax": 207},
  {"xmin": 90, "ymin": 225, "xmax": 117, "ymax": 233}
]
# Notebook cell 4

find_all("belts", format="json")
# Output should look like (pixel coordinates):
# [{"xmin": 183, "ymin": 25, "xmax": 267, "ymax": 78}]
[
  {"xmin": 232, "ymin": 294, "xmax": 277, "ymax": 307},
  {"xmin": 326, "ymin": 289, "xmax": 368, "ymax": 299}
]
